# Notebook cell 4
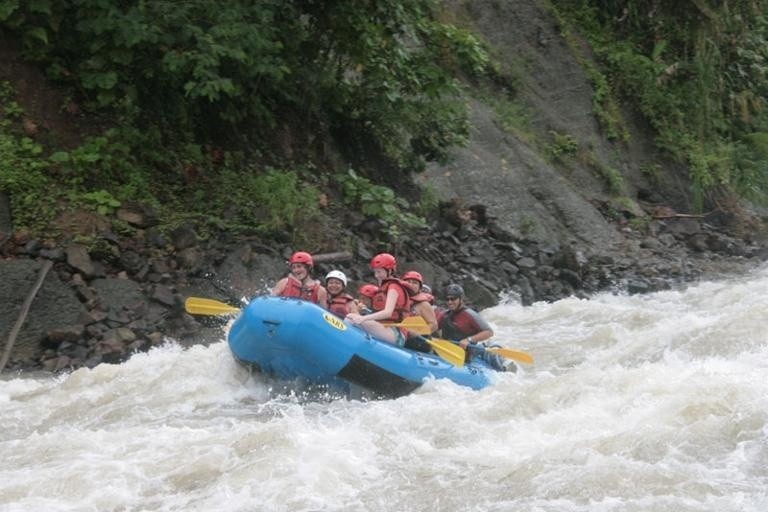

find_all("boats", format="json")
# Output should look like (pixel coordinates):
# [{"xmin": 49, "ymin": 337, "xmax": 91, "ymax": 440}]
[{"xmin": 225, "ymin": 296, "xmax": 509, "ymax": 396}]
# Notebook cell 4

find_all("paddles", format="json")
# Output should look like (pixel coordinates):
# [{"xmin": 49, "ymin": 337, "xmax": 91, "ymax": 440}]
[
  {"xmin": 449, "ymin": 339, "xmax": 534, "ymax": 365},
  {"xmin": 365, "ymin": 308, "xmax": 467, "ymax": 367},
  {"xmin": 185, "ymin": 296, "xmax": 242, "ymax": 316},
  {"xmin": 384, "ymin": 317, "xmax": 431, "ymax": 336}
]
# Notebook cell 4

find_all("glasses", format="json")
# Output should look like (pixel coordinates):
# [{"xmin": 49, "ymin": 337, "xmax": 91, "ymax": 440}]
[{"xmin": 444, "ymin": 295, "xmax": 459, "ymax": 301}]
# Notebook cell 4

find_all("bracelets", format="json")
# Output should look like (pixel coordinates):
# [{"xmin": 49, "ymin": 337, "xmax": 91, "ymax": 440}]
[{"xmin": 467, "ymin": 336, "xmax": 476, "ymax": 344}]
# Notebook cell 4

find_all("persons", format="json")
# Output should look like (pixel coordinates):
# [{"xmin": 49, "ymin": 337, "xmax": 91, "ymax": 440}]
[
  {"xmin": 323, "ymin": 269, "xmax": 356, "ymax": 317},
  {"xmin": 399, "ymin": 269, "xmax": 439, "ymax": 353},
  {"xmin": 270, "ymin": 251, "xmax": 329, "ymax": 309},
  {"xmin": 356, "ymin": 284, "xmax": 380, "ymax": 308},
  {"xmin": 420, "ymin": 283, "xmax": 444, "ymax": 328},
  {"xmin": 436, "ymin": 283, "xmax": 493, "ymax": 356},
  {"xmin": 344, "ymin": 253, "xmax": 410, "ymax": 345}
]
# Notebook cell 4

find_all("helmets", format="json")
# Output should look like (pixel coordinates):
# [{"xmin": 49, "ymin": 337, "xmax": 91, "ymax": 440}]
[
  {"xmin": 289, "ymin": 251, "xmax": 313, "ymax": 269},
  {"xmin": 325, "ymin": 269, "xmax": 347, "ymax": 287},
  {"xmin": 370, "ymin": 253, "xmax": 397, "ymax": 269},
  {"xmin": 358, "ymin": 284, "xmax": 379, "ymax": 299},
  {"xmin": 400, "ymin": 270, "xmax": 432, "ymax": 294},
  {"xmin": 444, "ymin": 284, "xmax": 464, "ymax": 304}
]
